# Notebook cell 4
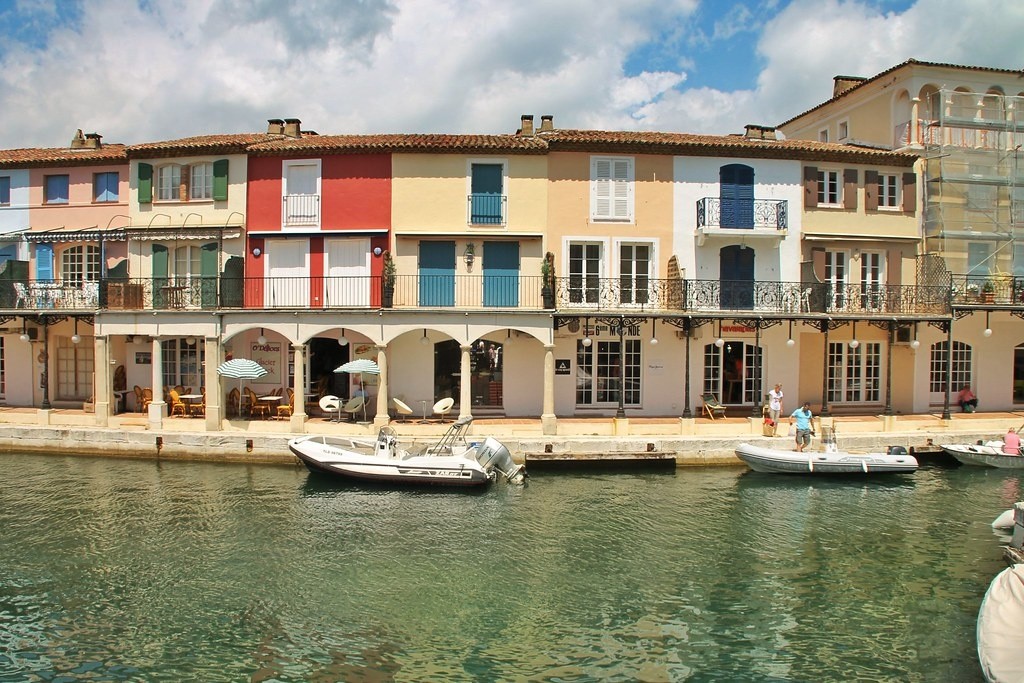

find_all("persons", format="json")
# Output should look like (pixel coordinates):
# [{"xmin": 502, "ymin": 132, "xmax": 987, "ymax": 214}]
[
  {"xmin": 730, "ymin": 354, "xmax": 751, "ymax": 381},
  {"xmin": 475, "ymin": 341, "xmax": 497, "ymax": 383},
  {"xmin": 767, "ymin": 382, "xmax": 784, "ymax": 437},
  {"xmin": 348, "ymin": 380, "xmax": 370, "ymax": 421},
  {"xmin": 958, "ymin": 384, "xmax": 979, "ymax": 413},
  {"xmin": 1001, "ymin": 427, "xmax": 1022, "ymax": 455},
  {"xmin": 789, "ymin": 402, "xmax": 816, "ymax": 453}
]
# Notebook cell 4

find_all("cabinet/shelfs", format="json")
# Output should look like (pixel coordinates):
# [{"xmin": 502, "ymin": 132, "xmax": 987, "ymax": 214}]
[{"xmin": 289, "ymin": 343, "xmax": 312, "ymax": 394}]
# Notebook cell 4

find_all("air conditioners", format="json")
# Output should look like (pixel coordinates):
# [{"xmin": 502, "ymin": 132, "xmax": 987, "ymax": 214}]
[
  {"xmin": 557, "ymin": 318, "xmax": 583, "ymax": 338},
  {"xmin": 26, "ymin": 325, "xmax": 46, "ymax": 342},
  {"xmin": 890, "ymin": 323, "xmax": 916, "ymax": 347},
  {"xmin": 675, "ymin": 319, "xmax": 703, "ymax": 341}
]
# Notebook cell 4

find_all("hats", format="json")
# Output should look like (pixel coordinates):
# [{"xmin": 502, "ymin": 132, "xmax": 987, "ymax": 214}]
[{"xmin": 1009, "ymin": 427, "xmax": 1015, "ymax": 432}]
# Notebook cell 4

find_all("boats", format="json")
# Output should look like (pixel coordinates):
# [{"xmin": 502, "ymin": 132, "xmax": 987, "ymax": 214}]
[
  {"xmin": 942, "ymin": 439, "xmax": 1023, "ymax": 467},
  {"xmin": 289, "ymin": 436, "xmax": 510, "ymax": 494},
  {"xmin": 731, "ymin": 445, "xmax": 921, "ymax": 474}
]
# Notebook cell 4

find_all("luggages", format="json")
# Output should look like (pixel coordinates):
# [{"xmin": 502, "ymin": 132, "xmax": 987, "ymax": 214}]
[{"xmin": 762, "ymin": 407, "xmax": 774, "ymax": 437}]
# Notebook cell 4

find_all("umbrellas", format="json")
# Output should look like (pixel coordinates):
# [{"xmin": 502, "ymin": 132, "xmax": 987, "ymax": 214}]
[
  {"xmin": 215, "ymin": 358, "xmax": 268, "ymax": 419},
  {"xmin": 332, "ymin": 358, "xmax": 383, "ymax": 423}
]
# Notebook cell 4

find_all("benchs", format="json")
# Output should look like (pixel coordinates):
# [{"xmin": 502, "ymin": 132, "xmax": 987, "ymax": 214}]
[{"xmin": 403, "ymin": 444, "xmax": 427, "ymax": 457}]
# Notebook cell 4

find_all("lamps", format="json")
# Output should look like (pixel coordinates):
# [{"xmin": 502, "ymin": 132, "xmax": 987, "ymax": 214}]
[
  {"xmin": 854, "ymin": 248, "xmax": 860, "ymax": 262},
  {"xmin": 252, "ymin": 247, "xmax": 261, "ymax": 257},
  {"xmin": 374, "ymin": 246, "xmax": 383, "ymax": 256},
  {"xmin": 20, "ymin": 310, "xmax": 995, "ymax": 349},
  {"xmin": 465, "ymin": 242, "xmax": 474, "ymax": 264},
  {"xmin": 740, "ymin": 236, "xmax": 747, "ymax": 250}
]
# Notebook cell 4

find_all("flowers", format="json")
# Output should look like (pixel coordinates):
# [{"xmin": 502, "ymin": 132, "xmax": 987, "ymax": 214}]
[
  {"xmin": 967, "ymin": 283, "xmax": 979, "ymax": 292},
  {"xmin": 945, "ymin": 284, "xmax": 964, "ymax": 298}
]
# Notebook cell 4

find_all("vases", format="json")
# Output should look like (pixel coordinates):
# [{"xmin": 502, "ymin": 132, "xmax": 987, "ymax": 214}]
[
  {"xmin": 954, "ymin": 295, "xmax": 963, "ymax": 304},
  {"xmin": 968, "ymin": 291, "xmax": 978, "ymax": 303}
]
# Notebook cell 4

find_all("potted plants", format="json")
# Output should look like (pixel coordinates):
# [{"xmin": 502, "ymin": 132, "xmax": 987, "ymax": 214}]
[
  {"xmin": 382, "ymin": 249, "xmax": 397, "ymax": 307},
  {"xmin": 541, "ymin": 251, "xmax": 556, "ymax": 308},
  {"xmin": 983, "ymin": 279, "xmax": 996, "ymax": 304}
]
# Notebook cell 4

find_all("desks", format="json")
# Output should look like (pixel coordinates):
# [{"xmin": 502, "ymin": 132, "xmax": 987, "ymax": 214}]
[
  {"xmin": 180, "ymin": 394, "xmax": 203, "ymax": 404},
  {"xmin": 241, "ymin": 394, "xmax": 263, "ymax": 414},
  {"xmin": 31, "ymin": 286, "xmax": 75, "ymax": 306},
  {"xmin": 162, "ymin": 286, "xmax": 189, "ymax": 310},
  {"xmin": 257, "ymin": 396, "xmax": 285, "ymax": 420},
  {"xmin": 304, "ymin": 392, "xmax": 319, "ymax": 417},
  {"xmin": 415, "ymin": 400, "xmax": 435, "ymax": 426},
  {"xmin": 330, "ymin": 398, "xmax": 350, "ymax": 425}
]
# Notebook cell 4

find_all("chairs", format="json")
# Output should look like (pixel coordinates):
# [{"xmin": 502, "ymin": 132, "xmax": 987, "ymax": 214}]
[
  {"xmin": 13, "ymin": 281, "xmax": 99, "ymax": 308},
  {"xmin": 699, "ymin": 393, "xmax": 728, "ymax": 421},
  {"xmin": 113, "ymin": 386, "xmax": 455, "ymax": 426},
  {"xmin": 763, "ymin": 395, "xmax": 769, "ymax": 419}
]
